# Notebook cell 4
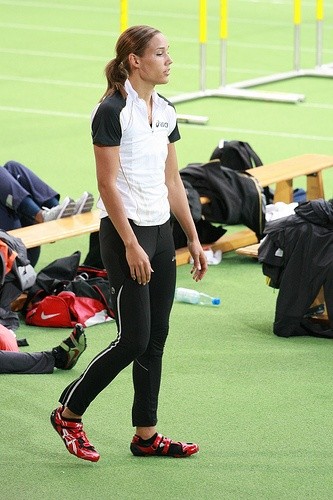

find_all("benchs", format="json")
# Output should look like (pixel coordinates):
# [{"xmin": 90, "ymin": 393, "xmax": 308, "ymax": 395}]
[{"xmin": 5, "ymin": 153, "xmax": 333, "ymax": 266}]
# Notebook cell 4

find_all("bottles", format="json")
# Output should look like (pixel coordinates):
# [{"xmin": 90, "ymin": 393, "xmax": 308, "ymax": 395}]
[{"xmin": 174, "ymin": 288, "xmax": 220, "ymax": 306}]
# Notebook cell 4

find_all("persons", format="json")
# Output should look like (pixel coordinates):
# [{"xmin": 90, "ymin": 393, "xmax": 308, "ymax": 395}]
[
  {"xmin": 0, "ymin": 324, "xmax": 87, "ymax": 375},
  {"xmin": 51, "ymin": 25, "xmax": 209, "ymax": 461},
  {"xmin": 0, "ymin": 161, "xmax": 94, "ymax": 268}
]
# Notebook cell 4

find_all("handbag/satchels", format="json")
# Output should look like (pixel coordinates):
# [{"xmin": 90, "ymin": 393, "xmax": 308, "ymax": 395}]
[{"xmin": 22, "ymin": 251, "xmax": 117, "ymax": 327}]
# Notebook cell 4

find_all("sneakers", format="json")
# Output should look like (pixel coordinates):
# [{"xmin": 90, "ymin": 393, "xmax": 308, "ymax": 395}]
[
  {"xmin": 73, "ymin": 191, "xmax": 94, "ymax": 216},
  {"xmin": 41, "ymin": 197, "xmax": 75, "ymax": 222},
  {"xmin": 51, "ymin": 406, "xmax": 99, "ymax": 462},
  {"xmin": 52, "ymin": 323, "xmax": 87, "ymax": 369},
  {"xmin": 130, "ymin": 432, "xmax": 199, "ymax": 458}
]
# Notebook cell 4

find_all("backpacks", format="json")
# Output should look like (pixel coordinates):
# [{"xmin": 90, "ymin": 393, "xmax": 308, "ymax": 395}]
[{"xmin": 210, "ymin": 139, "xmax": 274, "ymax": 205}]
